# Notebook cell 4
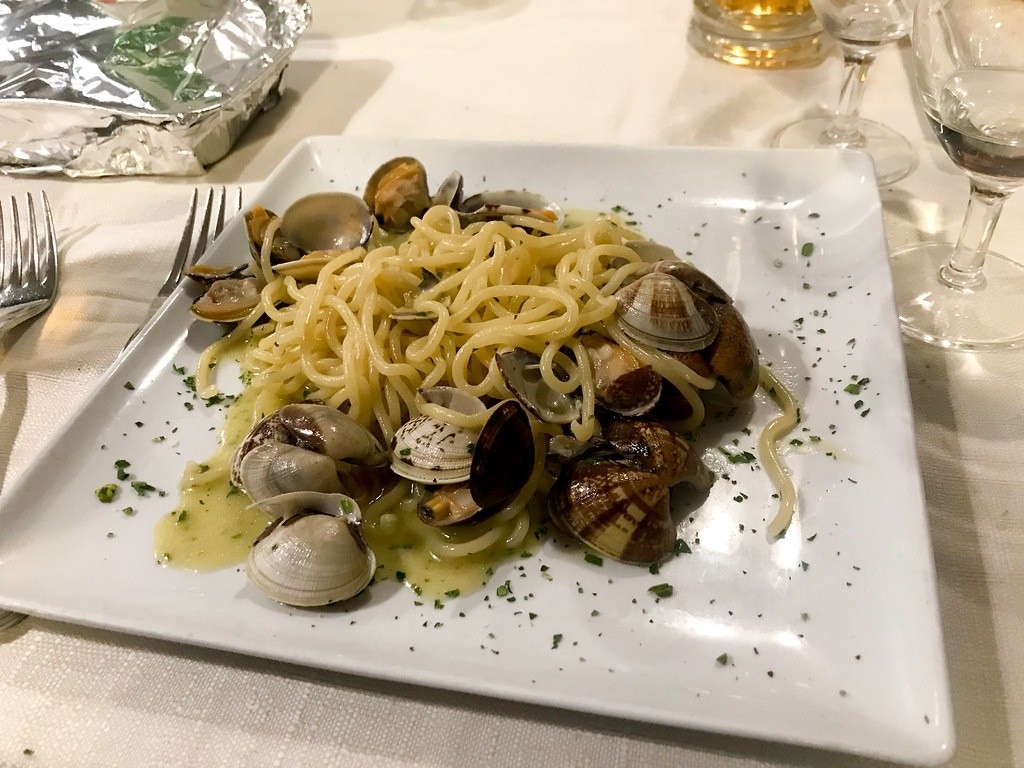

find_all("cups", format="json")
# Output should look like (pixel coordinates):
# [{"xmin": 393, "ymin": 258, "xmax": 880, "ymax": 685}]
[{"xmin": 685, "ymin": 0, "xmax": 836, "ymax": 69}]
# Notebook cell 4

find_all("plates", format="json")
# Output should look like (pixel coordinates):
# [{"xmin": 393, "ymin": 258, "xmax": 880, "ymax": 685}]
[{"xmin": 0, "ymin": 133, "xmax": 957, "ymax": 768}]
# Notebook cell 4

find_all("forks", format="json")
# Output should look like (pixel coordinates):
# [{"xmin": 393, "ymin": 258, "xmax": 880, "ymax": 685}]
[
  {"xmin": 0, "ymin": 190, "xmax": 60, "ymax": 339},
  {"xmin": 0, "ymin": 184, "xmax": 245, "ymax": 631}
]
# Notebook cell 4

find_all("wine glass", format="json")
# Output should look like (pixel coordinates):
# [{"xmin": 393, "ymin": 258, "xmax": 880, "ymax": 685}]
[
  {"xmin": 886, "ymin": 0, "xmax": 1024, "ymax": 350},
  {"xmin": 770, "ymin": 0, "xmax": 950, "ymax": 188}
]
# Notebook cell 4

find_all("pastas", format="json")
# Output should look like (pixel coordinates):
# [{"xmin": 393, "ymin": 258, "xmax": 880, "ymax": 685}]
[{"xmin": 176, "ymin": 206, "xmax": 803, "ymax": 540}]
